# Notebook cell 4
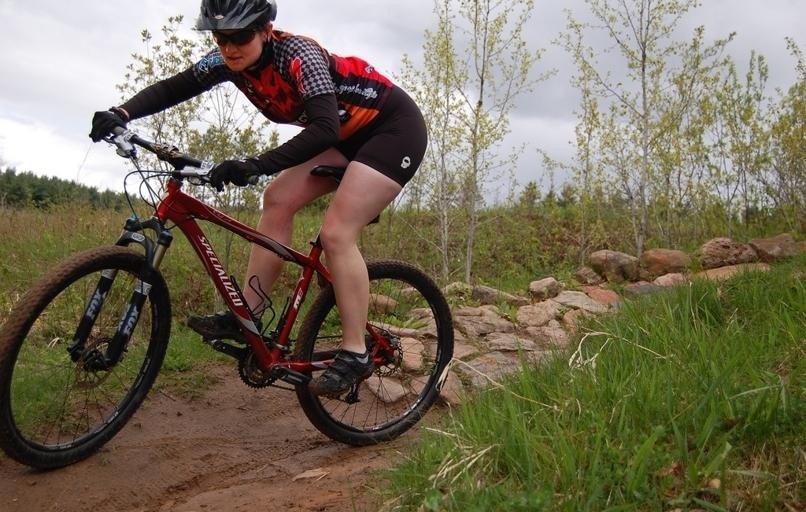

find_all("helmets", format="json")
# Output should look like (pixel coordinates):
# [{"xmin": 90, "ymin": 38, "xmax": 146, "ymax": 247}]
[{"xmin": 197, "ymin": 0, "xmax": 276, "ymax": 31}]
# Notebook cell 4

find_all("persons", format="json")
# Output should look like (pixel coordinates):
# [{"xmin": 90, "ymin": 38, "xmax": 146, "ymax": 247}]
[{"xmin": 91, "ymin": 0, "xmax": 427, "ymax": 396}]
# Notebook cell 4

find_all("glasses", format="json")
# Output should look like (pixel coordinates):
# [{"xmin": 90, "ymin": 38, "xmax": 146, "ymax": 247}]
[{"xmin": 213, "ymin": 30, "xmax": 254, "ymax": 44}]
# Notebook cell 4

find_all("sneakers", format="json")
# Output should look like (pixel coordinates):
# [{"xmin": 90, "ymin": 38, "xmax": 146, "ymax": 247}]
[
  {"xmin": 308, "ymin": 351, "xmax": 376, "ymax": 394},
  {"xmin": 188, "ymin": 309, "xmax": 262, "ymax": 341}
]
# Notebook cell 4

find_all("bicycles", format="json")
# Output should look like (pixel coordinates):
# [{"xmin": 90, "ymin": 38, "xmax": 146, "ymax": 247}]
[{"xmin": 1, "ymin": 125, "xmax": 453, "ymax": 471}]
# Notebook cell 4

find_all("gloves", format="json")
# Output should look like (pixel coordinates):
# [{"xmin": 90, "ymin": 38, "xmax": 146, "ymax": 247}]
[
  {"xmin": 89, "ymin": 107, "xmax": 128, "ymax": 142},
  {"xmin": 211, "ymin": 159, "xmax": 264, "ymax": 191}
]
